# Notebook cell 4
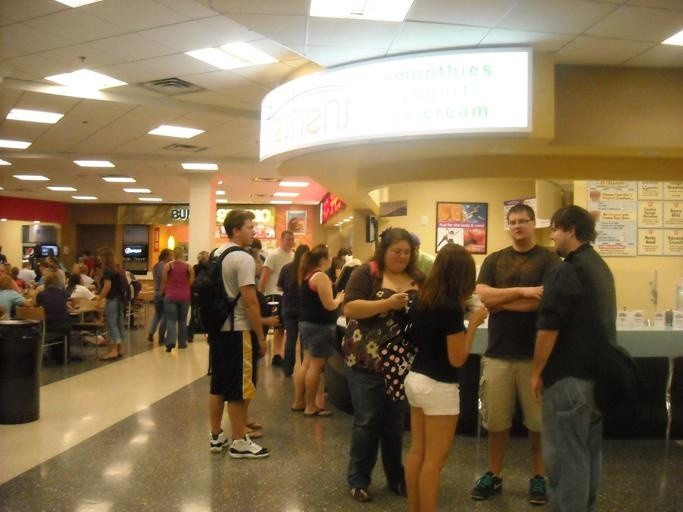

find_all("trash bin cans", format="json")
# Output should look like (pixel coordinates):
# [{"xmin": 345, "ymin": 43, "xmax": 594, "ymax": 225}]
[{"xmin": 1, "ymin": 318, "xmax": 42, "ymax": 426}]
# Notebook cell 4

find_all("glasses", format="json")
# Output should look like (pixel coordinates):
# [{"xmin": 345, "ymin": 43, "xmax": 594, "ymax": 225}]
[{"xmin": 508, "ymin": 218, "xmax": 530, "ymax": 225}]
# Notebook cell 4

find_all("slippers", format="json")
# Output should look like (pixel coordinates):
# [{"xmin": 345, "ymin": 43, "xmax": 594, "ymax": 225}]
[
  {"xmin": 247, "ymin": 422, "xmax": 262, "ymax": 429},
  {"xmin": 303, "ymin": 409, "xmax": 333, "ymax": 417},
  {"xmin": 292, "ymin": 407, "xmax": 305, "ymax": 411},
  {"xmin": 247, "ymin": 430, "xmax": 262, "ymax": 438}
]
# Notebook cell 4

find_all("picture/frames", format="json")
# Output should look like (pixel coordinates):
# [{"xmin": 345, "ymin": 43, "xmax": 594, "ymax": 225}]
[
  {"xmin": 286, "ymin": 210, "xmax": 307, "ymax": 236},
  {"xmin": 366, "ymin": 216, "xmax": 375, "ymax": 242},
  {"xmin": 435, "ymin": 201, "xmax": 488, "ymax": 254}
]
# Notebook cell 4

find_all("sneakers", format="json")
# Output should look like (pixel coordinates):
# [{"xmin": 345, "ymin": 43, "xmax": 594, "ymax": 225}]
[
  {"xmin": 350, "ymin": 488, "xmax": 370, "ymax": 502},
  {"xmin": 209, "ymin": 430, "xmax": 229, "ymax": 453},
  {"xmin": 271, "ymin": 355, "xmax": 283, "ymax": 367},
  {"xmin": 528, "ymin": 473, "xmax": 547, "ymax": 504},
  {"xmin": 228, "ymin": 433, "xmax": 272, "ymax": 458},
  {"xmin": 391, "ymin": 482, "xmax": 406, "ymax": 497},
  {"xmin": 470, "ymin": 471, "xmax": 503, "ymax": 500}
]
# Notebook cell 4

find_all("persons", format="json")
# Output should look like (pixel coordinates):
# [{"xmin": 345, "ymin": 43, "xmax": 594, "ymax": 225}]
[
  {"xmin": 148, "ymin": 248, "xmax": 175, "ymax": 345},
  {"xmin": 0, "ymin": 246, "xmax": 142, "ymax": 370},
  {"xmin": 291, "ymin": 243, "xmax": 346, "ymax": 416},
  {"xmin": 402, "ymin": 243, "xmax": 490, "ymax": 511},
  {"xmin": 315, "ymin": 257, "xmax": 346, "ymax": 416},
  {"xmin": 437, "ymin": 224, "xmax": 460, "ymax": 247},
  {"xmin": 276, "ymin": 244, "xmax": 311, "ymax": 379},
  {"xmin": 257, "ymin": 231, "xmax": 296, "ymax": 367},
  {"xmin": 207, "ymin": 209, "xmax": 272, "ymax": 458},
  {"xmin": 469, "ymin": 203, "xmax": 563, "ymax": 504},
  {"xmin": 244, "ymin": 238, "xmax": 279, "ymax": 439},
  {"xmin": 187, "ymin": 251, "xmax": 210, "ymax": 342},
  {"xmin": 407, "ymin": 234, "xmax": 437, "ymax": 282},
  {"xmin": 527, "ymin": 204, "xmax": 617, "ymax": 512},
  {"xmin": 158, "ymin": 246, "xmax": 195, "ymax": 352},
  {"xmin": 340, "ymin": 228, "xmax": 421, "ymax": 502},
  {"xmin": 338, "ymin": 248, "xmax": 361, "ymax": 268}
]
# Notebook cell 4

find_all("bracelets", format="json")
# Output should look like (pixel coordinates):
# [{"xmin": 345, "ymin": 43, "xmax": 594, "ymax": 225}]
[{"xmin": 256, "ymin": 338, "xmax": 267, "ymax": 343}]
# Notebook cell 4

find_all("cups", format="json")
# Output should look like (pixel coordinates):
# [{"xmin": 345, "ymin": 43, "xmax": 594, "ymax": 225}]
[
  {"xmin": 464, "ymin": 295, "xmax": 482, "ymax": 311},
  {"xmin": 267, "ymin": 301, "xmax": 280, "ymax": 316}
]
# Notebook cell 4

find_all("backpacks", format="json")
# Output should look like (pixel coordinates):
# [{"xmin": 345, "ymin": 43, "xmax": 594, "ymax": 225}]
[{"xmin": 191, "ymin": 245, "xmax": 247, "ymax": 335}]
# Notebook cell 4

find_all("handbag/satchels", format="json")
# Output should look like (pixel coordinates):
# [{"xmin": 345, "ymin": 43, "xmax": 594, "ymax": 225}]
[
  {"xmin": 593, "ymin": 346, "xmax": 646, "ymax": 435},
  {"xmin": 377, "ymin": 333, "xmax": 414, "ymax": 404}
]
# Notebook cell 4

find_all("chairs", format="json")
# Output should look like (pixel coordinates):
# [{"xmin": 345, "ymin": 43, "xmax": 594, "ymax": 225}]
[{"xmin": 0, "ymin": 281, "xmax": 141, "ymax": 367}]
